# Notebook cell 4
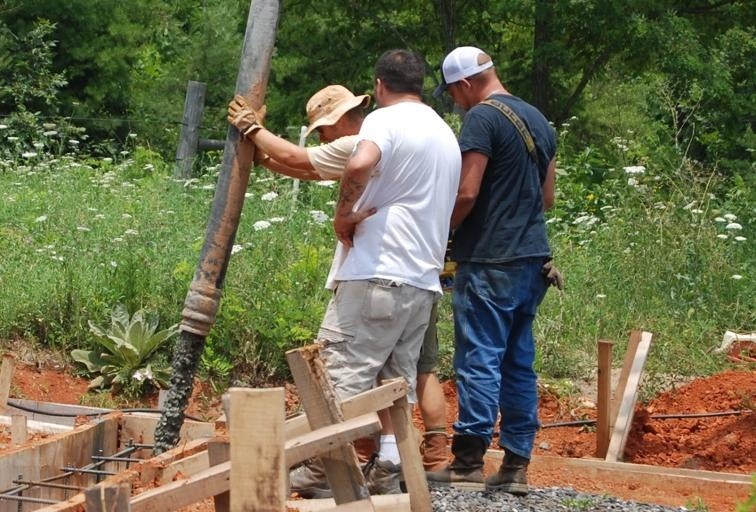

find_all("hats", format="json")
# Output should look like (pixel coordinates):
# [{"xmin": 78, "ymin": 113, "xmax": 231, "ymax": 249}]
[
  {"xmin": 431, "ymin": 45, "xmax": 495, "ymax": 99},
  {"xmin": 303, "ymin": 83, "xmax": 371, "ymax": 140}
]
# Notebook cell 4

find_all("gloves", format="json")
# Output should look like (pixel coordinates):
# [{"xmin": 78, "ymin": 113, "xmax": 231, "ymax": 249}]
[{"xmin": 226, "ymin": 93, "xmax": 270, "ymax": 136}]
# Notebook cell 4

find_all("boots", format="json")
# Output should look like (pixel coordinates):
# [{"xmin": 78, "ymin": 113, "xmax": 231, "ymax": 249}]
[
  {"xmin": 486, "ymin": 450, "xmax": 530, "ymax": 495},
  {"xmin": 361, "ymin": 453, "xmax": 404, "ymax": 493},
  {"xmin": 419, "ymin": 431, "xmax": 485, "ymax": 490},
  {"xmin": 287, "ymin": 456, "xmax": 332, "ymax": 499}
]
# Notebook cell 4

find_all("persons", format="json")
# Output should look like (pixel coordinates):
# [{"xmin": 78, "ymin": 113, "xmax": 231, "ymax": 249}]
[
  {"xmin": 431, "ymin": 44, "xmax": 563, "ymax": 494},
  {"xmin": 281, "ymin": 51, "xmax": 466, "ymax": 501},
  {"xmin": 224, "ymin": 82, "xmax": 456, "ymax": 486}
]
